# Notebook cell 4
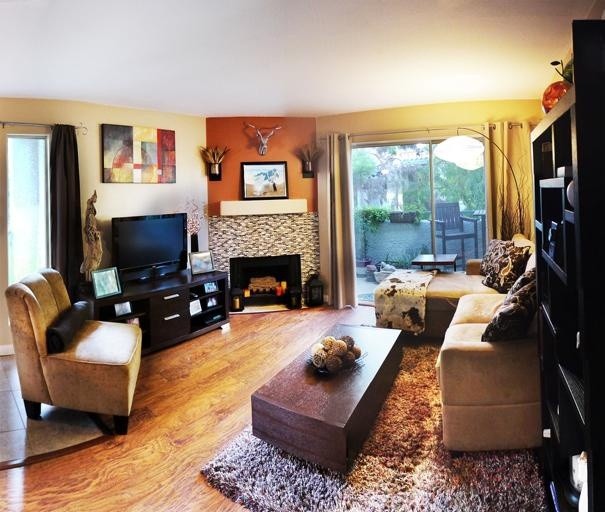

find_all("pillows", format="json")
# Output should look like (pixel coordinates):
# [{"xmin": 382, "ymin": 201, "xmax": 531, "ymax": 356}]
[
  {"xmin": 480, "ymin": 238, "xmax": 515, "ymax": 278},
  {"xmin": 505, "ymin": 266, "xmax": 536, "ymax": 302},
  {"xmin": 481, "ymin": 243, "xmax": 531, "ymax": 294},
  {"xmin": 44, "ymin": 300, "xmax": 91, "ymax": 356},
  {"xmin": 481, "ymin": 279, "xmax": 542, "ymax": 343}
]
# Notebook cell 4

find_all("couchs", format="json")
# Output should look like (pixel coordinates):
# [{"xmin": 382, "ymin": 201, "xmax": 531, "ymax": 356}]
[
  {"xmin": 4, "ymin": 267, "xmax": 142, "ymax": 436},
  {"xmin": 374, "ymin": 232, "xmax": 536, "ymax": 342},
  {"xmin": 434, "ymin": 248, "xmax": 544, "ymax": 456}
]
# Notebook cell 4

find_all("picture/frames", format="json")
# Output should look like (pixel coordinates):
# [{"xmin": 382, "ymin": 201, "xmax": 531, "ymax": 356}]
[
  {"xmin": 188, "ymin": 250, "xmax": 215, "ymax": 276},
  {"xmin": 90, "ymin": 267, "xmax": 123, "ymax": 299},
  {"xmin": 203, "ymin": 280, "xmax": 219, "ymax": 295},
  {"xmin": 240, "ymin": 161, "xmax": 289, "ymax": 201}
]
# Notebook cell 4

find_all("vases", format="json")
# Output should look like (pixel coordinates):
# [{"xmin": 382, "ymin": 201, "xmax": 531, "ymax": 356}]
[
  {"xmin": 403, "ymin": 212, "xmax": 416, "ymax": 222},
  {"xmin": 387, "ymin": 212, "xmax": 403, "ymax": 223}
]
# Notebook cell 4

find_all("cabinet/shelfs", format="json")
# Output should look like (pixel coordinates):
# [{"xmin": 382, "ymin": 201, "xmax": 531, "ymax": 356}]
[
  {"xmin": 526, "ymin": 19, "xmax": 605, "ymax": 512},
  {"xmin": 77, "ymin": 267, "xmax": 231, "ymax": 359}
]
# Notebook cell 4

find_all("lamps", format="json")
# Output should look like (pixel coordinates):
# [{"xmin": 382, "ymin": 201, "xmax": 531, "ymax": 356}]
[{"xmin": 432, "ymin": 128, "xmax": 524, "ymax": 235}]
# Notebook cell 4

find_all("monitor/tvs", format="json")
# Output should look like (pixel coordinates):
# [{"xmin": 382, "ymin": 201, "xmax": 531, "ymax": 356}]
[{"xmin": 111, "ymin": 212, "xmax": 189, "ymax": 274}]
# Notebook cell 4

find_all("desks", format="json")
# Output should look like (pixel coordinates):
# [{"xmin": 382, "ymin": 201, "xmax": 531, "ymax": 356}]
[{"xmin": 473, "ymin": 209, "xmax": 487, "ymax": 258}]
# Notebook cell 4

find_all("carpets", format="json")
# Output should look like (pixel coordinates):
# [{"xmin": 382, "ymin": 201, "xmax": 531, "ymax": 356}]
[
  {"xmin": 199, "ymin": 336, "xmax": 547, "ymax": 512},
  {"xmin": 0, "ymin": 352, "xmax": 116, "ymax": 471}
]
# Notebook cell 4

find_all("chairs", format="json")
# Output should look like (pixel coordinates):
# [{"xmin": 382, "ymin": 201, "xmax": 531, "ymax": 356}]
[{"xmin": 431, "ymin": 201, "xmax": 481, "ymax": 271}]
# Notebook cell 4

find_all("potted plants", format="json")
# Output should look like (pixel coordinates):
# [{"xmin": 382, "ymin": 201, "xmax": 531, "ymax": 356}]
[
  {"xmin": 184, "ymin": 197, "xmax": 208, "ymax": 253},
  {"xmin": 356, "ymin": 220, "xmax": 373, "ymax": 267},
  {"xmin": 202, "ymin": 145, "xmax": 230, "ymax": 175},
  {"xmin": 294, "ymin": 142, "xmax": 325, "ymax": 171}
]
